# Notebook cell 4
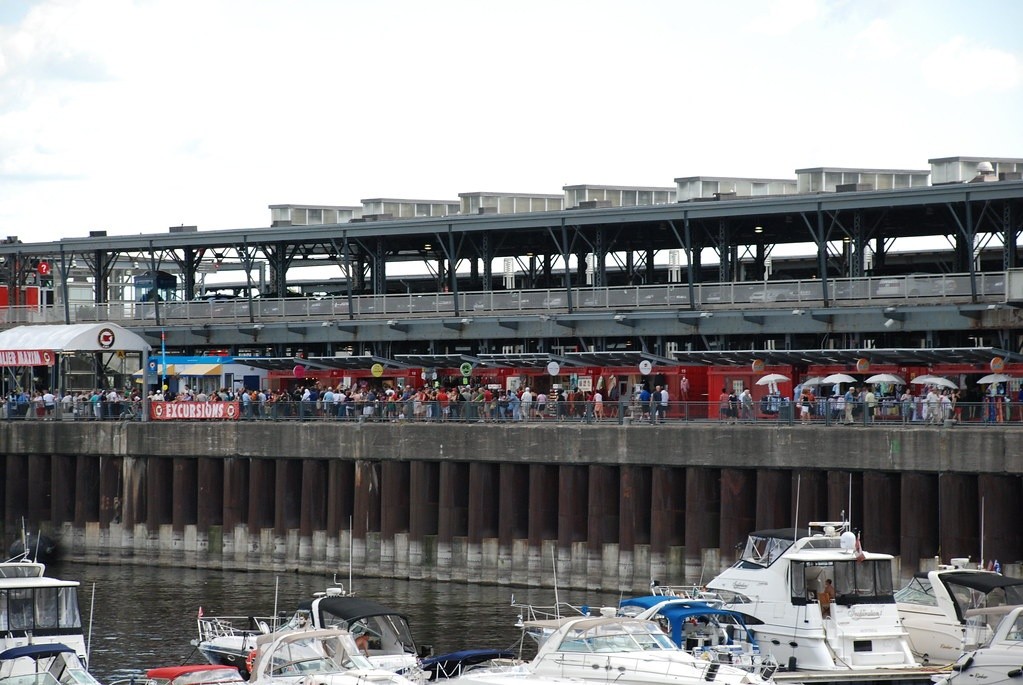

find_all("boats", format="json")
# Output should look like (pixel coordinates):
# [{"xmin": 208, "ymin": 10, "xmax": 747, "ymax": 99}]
[
  {"xmin": 421, "ymin": 474, "xmax": 1023, "ymax": 684},
  {"xmin": 0, "ymin": 471, "xmax": 433, "ymax": 685}
]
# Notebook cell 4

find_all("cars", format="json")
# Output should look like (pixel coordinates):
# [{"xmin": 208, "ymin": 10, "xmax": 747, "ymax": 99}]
[
  {"xmin": 877, "ymin": 272, "xmax": 957, "ymax": 297},
  {"xmin": 748, "ymin": 285, "xmax": 876, "ymax": 303},
  {"xmin": 431, "ymin": 285, "xmax": 750, "ymax": 314},
  {"xmin": 170, "ymin": 290, "xmax": 438, "ymax": 318}
]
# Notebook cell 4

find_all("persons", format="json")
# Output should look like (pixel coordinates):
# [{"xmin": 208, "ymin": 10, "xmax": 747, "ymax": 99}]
[
  {"xmin": 824, "ymin": 579, "xmax": 835, "ymax": 599},
  {"xmin": 1, "ymin": 382, "xmax": 671, "ymax": 425},
  {"xmin": 718, "ymin": 383, "xmax": 1023, "ymax": 425},
  {"xmin": 356, "ymin": 632, "xmax": 371, "ymax": 657}
]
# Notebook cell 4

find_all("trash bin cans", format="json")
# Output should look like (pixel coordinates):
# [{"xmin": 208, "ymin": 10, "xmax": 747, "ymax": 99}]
[{"xmin": 777, "ymin": 406, "xmax": 789, "ymax": 421}]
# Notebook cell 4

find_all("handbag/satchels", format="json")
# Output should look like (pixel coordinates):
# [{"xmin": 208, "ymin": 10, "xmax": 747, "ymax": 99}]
[{"xmin": 797, "ymin": 394, "xmax": 804, "ymax": 408}]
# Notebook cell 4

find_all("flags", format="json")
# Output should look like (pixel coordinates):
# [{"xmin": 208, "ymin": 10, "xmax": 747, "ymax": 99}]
[
  {"xmin": 198, "ymin": 606, "xmax": 204, "ymax": 617},
  {"xmin": 853, "ymin": 532, "xmax": 865, "ymax": 565}
]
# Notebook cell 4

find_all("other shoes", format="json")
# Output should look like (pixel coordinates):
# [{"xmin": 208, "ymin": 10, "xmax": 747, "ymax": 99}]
[{"xmin": 660, "ymin": 421, "xmax": 666, "ymax": 423}]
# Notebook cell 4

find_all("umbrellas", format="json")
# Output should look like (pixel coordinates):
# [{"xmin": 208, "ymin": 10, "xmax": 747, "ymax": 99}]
[
  {"xmin": 756, "ymin": 373, "xmax": 791, "ymax": 386},
  {"xmin": 977, "ymin": 373, "xmax": 1011, "ymax": 384},
  {"xmin": 821, "ymin": 372, "xmax": 857, "ymax": 383},
  {"xmin": 804, "ymin": 377, "xmax": 826, "ymax": 385},
  {"xmin": 864, "ymin": 373, "xmax": 906, "ymax": 386},
  {"xmin": 925, "ymin": 377, "xmax": 959, "ymax": 389},
  {"xmin": 910, "ymin": 374, "xmax": 940, "ymax": 387}
]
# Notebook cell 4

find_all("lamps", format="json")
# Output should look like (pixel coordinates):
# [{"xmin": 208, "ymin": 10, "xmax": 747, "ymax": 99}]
[
  {"xmin": 387, "ymin": 321, "xmax": 399, "ymax": 326},
  {"xmin": 613, "ymin": 315, "xmax": 626, "ymax": 322},
  {"xmin": 460, "ymin": 319, "xmax": 474, "ymax": 325},
  {"xmin": 252, "ymin": 325, "xmax": 265, "ymax": 330},
  {"xmin": 539, "ymin": 316, "xmax": 551, "ymax": 322},
  {"xmin": 321, "ymin": 322, "xmax": 333, "ymax": 328},
  {"xmin": 792, "ymin": 310, "xmax": 806, "ymax": 316},
  {"xmin": 700, "ymin": 313, "xmax": 713, "ymax": 319},
  {"xmin": 986, "ymin": 304, "xmax": 1002, "ymax": 313}
]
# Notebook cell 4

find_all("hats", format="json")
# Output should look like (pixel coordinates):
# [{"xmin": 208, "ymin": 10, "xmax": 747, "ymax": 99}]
[
  {"xmin": 849, "ymin": 387, "xmax": 855, "ymax": 390},
  {"xmin": 328, "ymin": 387, "xmax": 332, "ymax": 391}
]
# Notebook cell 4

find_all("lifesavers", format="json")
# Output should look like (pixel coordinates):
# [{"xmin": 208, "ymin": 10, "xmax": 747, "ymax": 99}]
[{"xmin": 247, "ymin": 650, "xmax": 257, "ymax": 673}]
